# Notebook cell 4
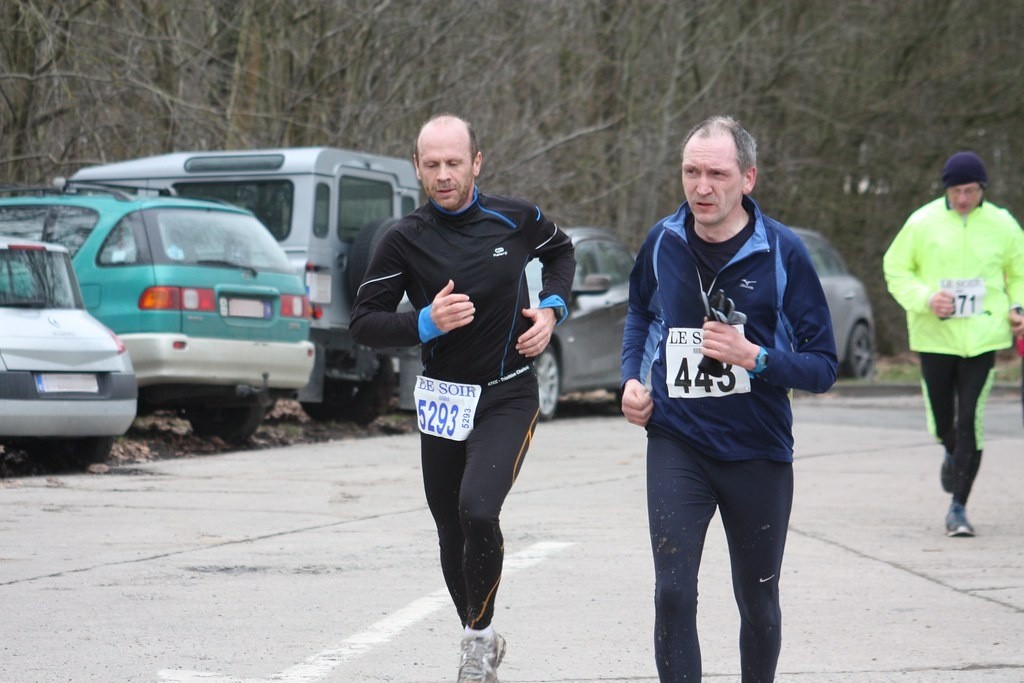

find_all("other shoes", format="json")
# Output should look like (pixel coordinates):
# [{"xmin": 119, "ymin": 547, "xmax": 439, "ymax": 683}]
[
  {"xmin": 457, "ymin": 630, "xmax": 501, "ymax": 683},
  {"xmin": 496, "ymin": 633, "xmax": 506, "ymax": 668}
]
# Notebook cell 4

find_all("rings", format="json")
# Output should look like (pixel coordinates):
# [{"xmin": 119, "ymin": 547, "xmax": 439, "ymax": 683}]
[{"xmin": 540, "ymin": 342, "xmax": 543, "ymax": 347}]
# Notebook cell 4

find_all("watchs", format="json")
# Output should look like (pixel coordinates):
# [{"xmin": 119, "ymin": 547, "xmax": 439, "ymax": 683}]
[
  {"xmin": 745, "ymin": 346, "xmax": 768, "ymax": 379},
  {"xmin": 553, "ymin": 306, "xmax": 565, "ymax": 321}
]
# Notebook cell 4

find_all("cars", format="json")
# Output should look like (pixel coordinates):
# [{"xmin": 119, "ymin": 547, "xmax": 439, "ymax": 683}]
[
  {"xmin": 0, "ymin": 235, "xmax": 138, "ymax": 471},
  {"xmin": 0, "ymin": 179, "xmax": 316, "ymax": 447},
  {"xmin": 523, "ymin": 227, "xmax": 642, "ymax": 421},
  {"xmin": 784, "ymin": 222, "xmax": 876, "ymax": 381}
]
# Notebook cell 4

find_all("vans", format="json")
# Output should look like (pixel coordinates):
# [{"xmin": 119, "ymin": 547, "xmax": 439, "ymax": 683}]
[{"xmin": 53, "ymin": 144, "xmax": 424, "ymax": 427}]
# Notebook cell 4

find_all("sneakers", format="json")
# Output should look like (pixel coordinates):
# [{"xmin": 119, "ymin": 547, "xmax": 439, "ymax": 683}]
[
  {"xmin": 940, "ymin": 451, "xmax": 954, "ymax": 493},
  {"xmin": 946, "ymin": 505, "xmax": 975, "ymax": 537}
]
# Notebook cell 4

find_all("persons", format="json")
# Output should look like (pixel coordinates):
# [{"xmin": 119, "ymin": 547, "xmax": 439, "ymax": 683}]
[
  {"xmin": 619, "ymin": 117, "xmax": 837, "ymax": 683},
  {"xmin": 883, "ymin": 150, "xmax": 1024, "ymax": 538},
  {"xmin": 348, "ymin": 113, "xmax": 577, "ymax": 683}
]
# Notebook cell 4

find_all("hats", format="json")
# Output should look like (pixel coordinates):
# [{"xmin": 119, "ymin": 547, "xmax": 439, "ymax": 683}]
[{"xmin": 941, "ymin": 151, "xmax": 988, "ymax": 188}]
